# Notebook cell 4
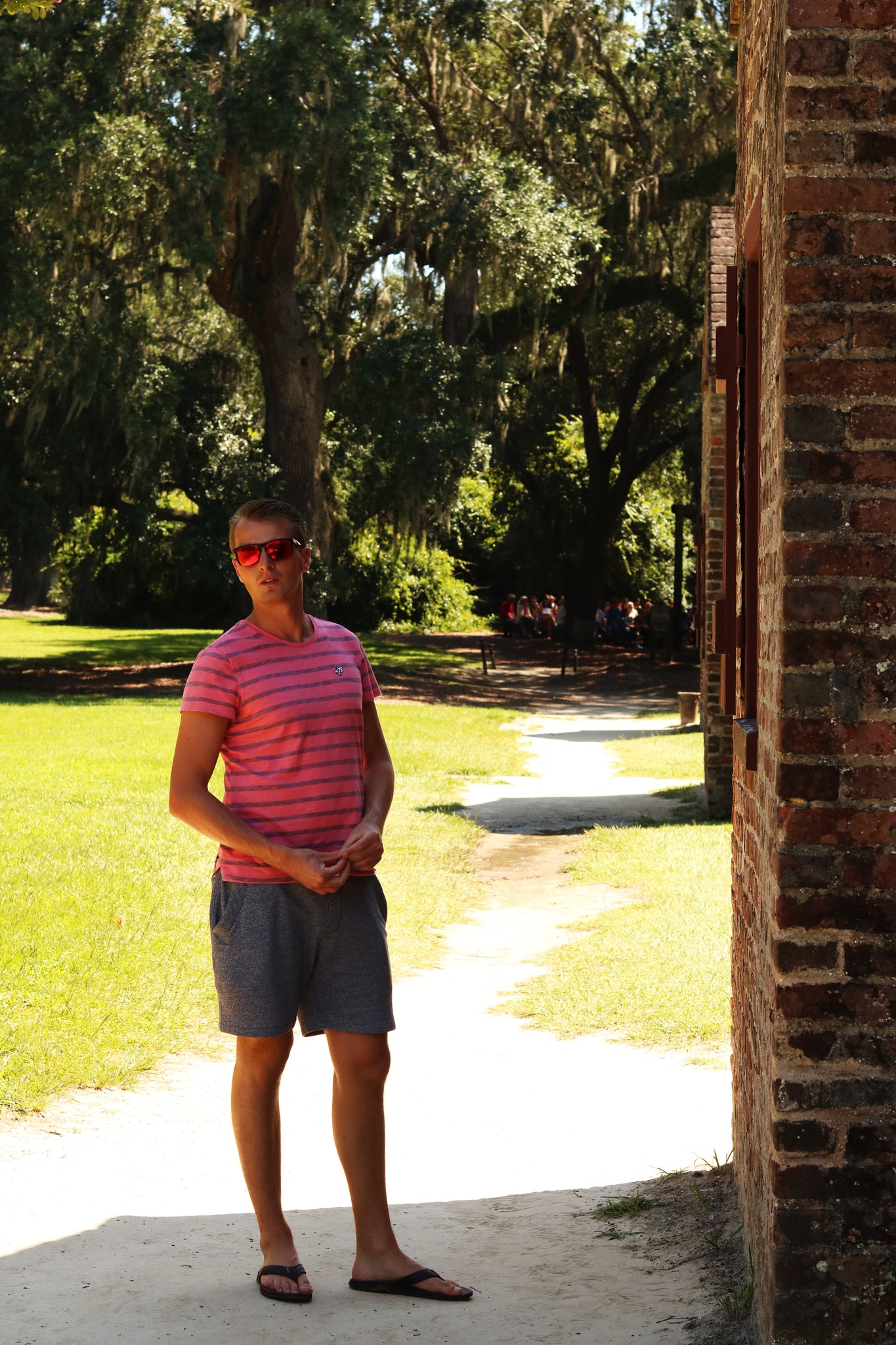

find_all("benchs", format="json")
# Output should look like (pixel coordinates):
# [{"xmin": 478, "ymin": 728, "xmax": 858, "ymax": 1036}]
[{"xmin": 482, "ymin": 634, "xmax": 577, "ymax": 675}]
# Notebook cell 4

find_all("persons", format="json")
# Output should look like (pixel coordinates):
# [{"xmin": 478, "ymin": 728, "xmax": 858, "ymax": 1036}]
[
  {"xmin": 498, "ymin": 593, "xmax": 696, "ymax": 648},
  {"xmin": 169, "ymin": 500, "xmax": 473, "ymax": 1302}
]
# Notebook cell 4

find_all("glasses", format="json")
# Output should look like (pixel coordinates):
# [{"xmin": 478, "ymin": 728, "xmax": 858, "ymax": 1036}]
[{"xmin": 233, "ymin": 537, "xmax": 302, "ymax": 567}]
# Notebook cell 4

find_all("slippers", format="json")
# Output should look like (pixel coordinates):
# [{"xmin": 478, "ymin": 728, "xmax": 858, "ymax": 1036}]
[
  {"xmin": 348, "ymin": 1268, "xmax": 474, "ymax": 1302},
  {"xmin": 257, "ymin": 1263, "xmax": 314, "ymax": 1304}
]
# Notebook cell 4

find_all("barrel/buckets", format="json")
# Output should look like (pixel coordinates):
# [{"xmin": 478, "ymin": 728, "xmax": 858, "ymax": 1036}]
[{"xmin": 677, "ymin": 691, "xmax": 700, "ymax": 724}]
